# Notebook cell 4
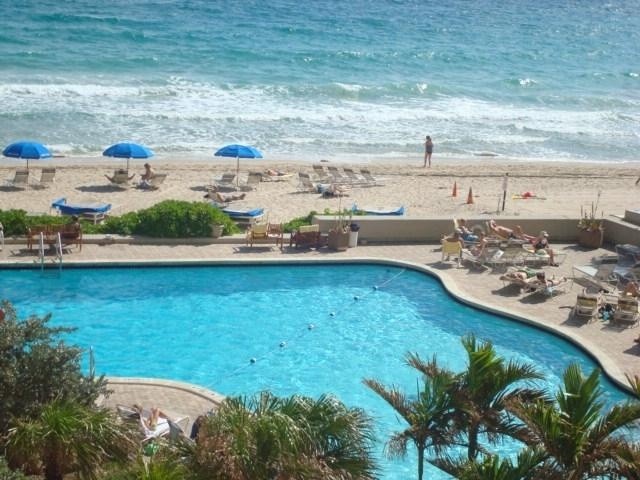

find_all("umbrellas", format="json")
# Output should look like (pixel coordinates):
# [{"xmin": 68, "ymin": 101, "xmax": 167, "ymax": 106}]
[
  {"xmin": 102, "ymin": 141, "xmax": 157, "ymax": 174},
  {"xmin": 215, "ymin": 143, "xmax": 263, "ymax": 187},
  {"xmin": 3, "ymin": 139, "xmax": 54, "ymax": 171}
]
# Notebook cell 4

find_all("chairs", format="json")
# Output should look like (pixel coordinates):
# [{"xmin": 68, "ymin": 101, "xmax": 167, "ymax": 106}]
[
  {"xmin": 4, "ymin": 167, "xmax": 57, "ymax": 192},
  {"xmin": 115, "ymin": 403, "xmax": 190, "ymax": 444},
  {"xmin": 112, "ymin": 169, "xmax": 167, "ymax": 192},
  {"xmin": 207, "ymin": 163, "xmax": 386, "ymax": 200},
  {"xmin": 439, "ymin": 215, "xmax": 640, "ymax": 328},
  {"xmin": 26, "ymin": 222, "xmax": 83, "ymax": 254},
  {"xmin": 245, "ymin": 223, "xmax": 322, "ymax": 252}
]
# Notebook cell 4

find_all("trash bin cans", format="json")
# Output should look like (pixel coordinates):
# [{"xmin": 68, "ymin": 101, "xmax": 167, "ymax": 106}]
[{"xmin": 348, "ymin": 223, "xmax": 359, "ymax": 248}]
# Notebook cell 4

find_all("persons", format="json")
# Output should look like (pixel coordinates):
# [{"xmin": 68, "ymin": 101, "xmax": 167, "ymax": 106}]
[
  {"xmin": 422, "ymin": 134, "xmax": 434, "ymax": 168},
  {"xmin": 446, "ymin": 215, "xmax": 640, "ymax": 325},
  {"xmin": 205, "ymin": 185, "xmax": 247, "ymax": 203},
  {"xmin": 104, "ymin": 169, "xmax": 137, "ymax": 184},
  {"xmin": 132, "ymin": 403, "xmax": 172, "ymax": 431},
  {"xmin": 139, "ymin": 163, "xmax": 157, "ymax": 184}
]
